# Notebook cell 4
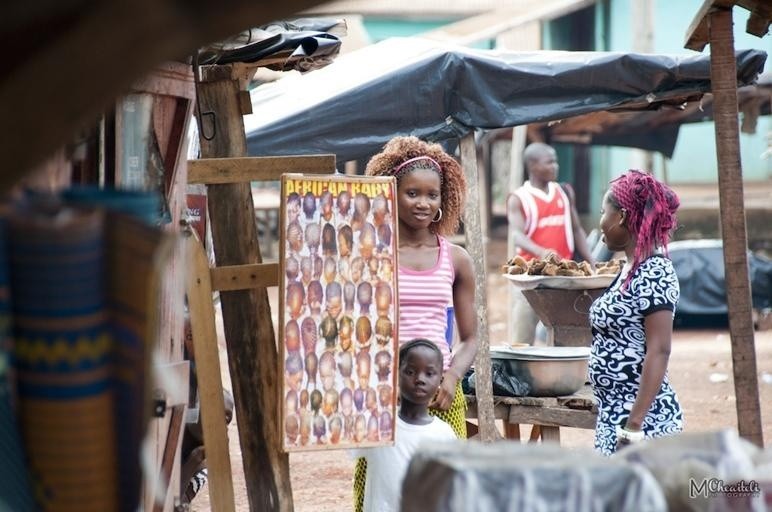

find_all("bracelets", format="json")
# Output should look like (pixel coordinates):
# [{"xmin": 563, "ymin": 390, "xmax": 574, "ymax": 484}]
[{"xmin": 614, "ymin": 424, "xmax": 646, "ymax": 443}]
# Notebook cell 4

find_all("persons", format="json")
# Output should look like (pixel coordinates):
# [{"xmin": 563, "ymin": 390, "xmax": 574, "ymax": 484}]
[
  {"xmin": 506, "ymin": 139, "xmax": 595, "ymax": 346},
  {"xmin": 350, "ymin": 133, "xmax": 482, "ymax": 512},
  {"xmin": 347, "ymin": 338, "xmax": 459, "ymax": 512},
  {"xmin": 586, "ymin": 167, "xmax": 684, "ymax": 456}
]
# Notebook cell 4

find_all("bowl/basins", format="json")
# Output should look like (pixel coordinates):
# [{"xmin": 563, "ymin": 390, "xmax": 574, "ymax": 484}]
[{"xmin": 487, "ymin": 342, "xmax": 594, "ymax": 398}]
